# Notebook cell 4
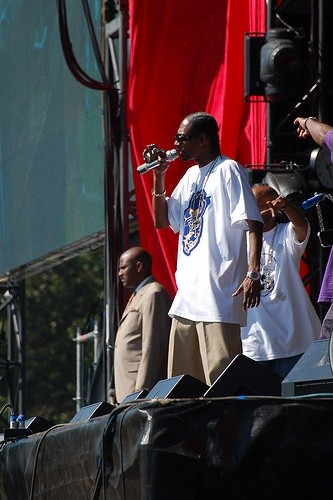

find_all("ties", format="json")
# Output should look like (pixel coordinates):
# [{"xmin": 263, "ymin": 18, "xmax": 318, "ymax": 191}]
[{"xmin": 128, "ymin": 291, "xmax": 136, "ymax": 306}]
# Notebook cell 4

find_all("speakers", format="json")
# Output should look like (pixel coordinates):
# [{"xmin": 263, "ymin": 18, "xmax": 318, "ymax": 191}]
[
  {"xmin": 67, "ymin": 339, "xmax": 333, "ymax": 424},
  {"xmin": 22, "ymin": 416, "xmax": 55, "ymax": 435}
]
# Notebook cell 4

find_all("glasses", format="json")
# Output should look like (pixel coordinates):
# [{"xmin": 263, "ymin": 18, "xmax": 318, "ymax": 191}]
[{"xmin": 175, "ymin": 134, "xmax": 202, "ymax": 143}]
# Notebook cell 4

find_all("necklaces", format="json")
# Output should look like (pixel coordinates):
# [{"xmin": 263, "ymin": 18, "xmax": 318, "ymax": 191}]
[{"xmin": 190, "ymin": 157, "xmax": 219, "ymax": 199}]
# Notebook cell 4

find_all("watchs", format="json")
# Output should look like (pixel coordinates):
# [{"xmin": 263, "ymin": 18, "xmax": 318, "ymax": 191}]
[
  {"xmin": 303, "ymin": 116, "xmax": 317, "ymax": 130},
  {"xmin": 245, "ymin": 271, "xmax": 261, "ymax": 280}
]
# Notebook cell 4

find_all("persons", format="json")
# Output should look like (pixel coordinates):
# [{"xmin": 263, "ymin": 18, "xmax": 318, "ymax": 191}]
[
  {"xmin": 294, "ymin": 117, "xmax": 333, "ymax": 341},
  {"xmin": 240, "ymin": 182, "xmax": 323, "ymax": 378},
  {"xmin": 144, "ymin": 112, "xmax": 265, "ymax": 386},
  {"xmin": 114, "ymin": 247, "xmax": 172, "ymax": 405}
]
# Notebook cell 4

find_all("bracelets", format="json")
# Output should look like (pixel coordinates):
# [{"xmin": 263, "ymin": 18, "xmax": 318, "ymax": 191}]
[
  {"xmin": 280, "ymin": 203, "xmax": 288, "ymax": 209},
  {"xmin": 150, "ymin": 189, "xmax": 167, "ymax": 197}
]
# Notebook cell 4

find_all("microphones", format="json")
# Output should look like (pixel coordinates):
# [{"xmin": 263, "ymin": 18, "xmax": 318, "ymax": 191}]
[{"xmin": 137, "ymin": 149, "xmax": 179, "ymax": 175}]
